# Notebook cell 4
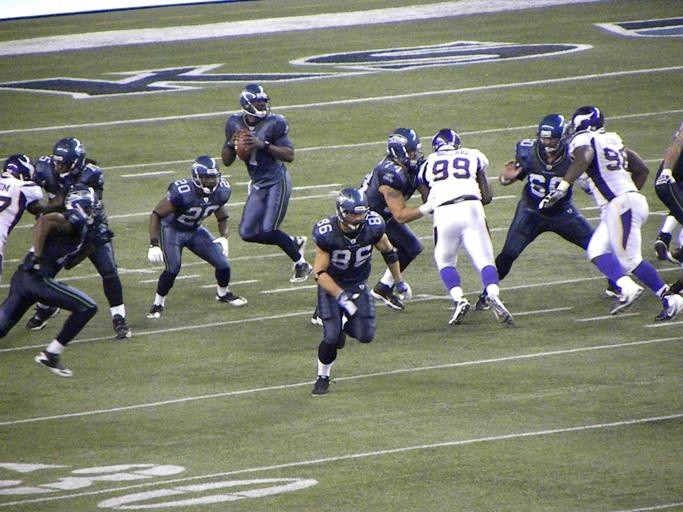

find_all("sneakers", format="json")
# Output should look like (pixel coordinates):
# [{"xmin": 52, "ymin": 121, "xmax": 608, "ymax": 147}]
[
  {"xmin": 475, "ymin": 287, "xmax": 493, "ymax": 312},
  {"xmin": 109, "ymin": 312, "xmax": 133, "ymax": 341},
  {"xmin": 610, "ymin": 286, "xmax": 645, "ymax": 316},
  {"xmin": 604, "ymin": 281, "xmax": 621, "ymax": 298},
  {"xmin": 289, "ymin": 233, "xmax": 308, "ymax": 259},
  {"xmin": 447, "ymin": 295, "xmax": 472, "ymax": 327},
  {"xmin": 335, "ymin": 328, "xmax": 348, "ymax": 351},
  {"xmin": 289, "ymin": 261, "xmax": 315, "ymax": 284},
  {"xmin": 369, "ymin": 280, "xmax": 406, "ymax": 313},
  {"xmin": 30, "ymin": 347, "xmax": 75, "ymax": 381},
  {"xmin": 653, "ymin": 233, "xmax": 672, "ymax": 261},
  {"xmin": 311, "ymin": 374, "xmax": 332, "ymax": 396},
  {"xmin": 666, "ymin": 245, "xmax": 683, "ymax": 270},
  {"xmin": 213, "ymin": 290, "xmax": 248, "ymax": 308},
  {"xmin": 484, "ymin": 295, "xmax": 519, "ymax": 330},
  {"xmin": 146, "ymin": 302, "xmax": 165, "ymax": 320},
  {"xmin": 23, "ymin": 304, "xmax": 63, "ymax": 331},
  {"xmin": 654, "ymin": 294, "xmax": 683, "ymax": 323},
  {"xmin": 310, "ymin": 307, "xmax": 324, "ymax": 327}
]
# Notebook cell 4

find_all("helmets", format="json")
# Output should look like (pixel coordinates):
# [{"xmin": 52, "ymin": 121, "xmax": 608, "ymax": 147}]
[
  {"xmin": 536, "ymin": 112, "xmax": 570, "ymax": 154},
  {"xmin": 334, "ymin": 185, "xmax": 370, "ymax": 233},
  {"xmin": 189, "ymin": 154, "xmax": 222, "ymax": 195},
  {"xmin": 430, "ymin": 127, "xmax": 462, "ymax": 152},
  {"xmin": 570, "ymin": 104, "xmax": 605, "ymax": 136},
  {"xmin": 50, "ymin": 135, "xmax": 87, "ymax": 182},
  {"xmin": 385, "ymin": 127, "xmax": 425, "ymax": 170},
  {"xmin": 2, "ymin": 152, "xmax": 37, "ymax": 183},
  {"xmin": 238, "ymin": 83, "xmax": 270, "ymax": 120},
  {"xmin": 64, "ymin": 184, "xmax": 103, "ymax": 227}
]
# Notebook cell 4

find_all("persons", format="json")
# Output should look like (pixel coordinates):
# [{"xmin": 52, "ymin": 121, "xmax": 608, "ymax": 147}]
[
  {"xmin": 476, "ymin": 113, "xmax": 622, "ymax": 309},
  {"xmin": 313, "ymin": 187, "xmax": 411, "ymax": 394},
  {"xmin": 222, "ymin": 83, "xmax": 314, "ymax": 283},
  {"xmin": 0, "ymin": 137, "xmax": 132, "ymax": 378},
  {"xmin": 360, "ymin": 129, "xmax": 424, "ymax": 311},
  {"xmin": 147, "ymin": 155, "xmax": 248, "ymax": 318},
  {"xmin": 654, "ymin": 125, "xmax": 683, "ymax": 263},
  {"xmin": 539, "ymin": 107, "xmax": 683, "ymax": 321},
  {"xmin": 416, "ymin": 129, "xmax": 512, "ymax": 326}
]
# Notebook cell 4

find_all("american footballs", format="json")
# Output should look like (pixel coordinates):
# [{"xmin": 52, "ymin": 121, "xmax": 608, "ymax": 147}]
[{"xmin": 235, "ymin": 129, "xmax": 256, "ymax": 162}]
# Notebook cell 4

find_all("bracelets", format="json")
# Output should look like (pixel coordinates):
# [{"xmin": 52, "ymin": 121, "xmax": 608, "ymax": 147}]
[
  {"xmin": 264, "ymin": 140, "xmax": 270, "ymax": 150},
  {"xmin": 150, "ymin": 237, "xmax": 160, "ymax": 247}
]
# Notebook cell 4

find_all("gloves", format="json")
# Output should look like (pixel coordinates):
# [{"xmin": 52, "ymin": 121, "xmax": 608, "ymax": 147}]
[
  {"xmin": 210, "ymin": 237, "xmax": 229, "ymax": 257},
  {"xmin": 654, "ymin": 168, "xmax": 681, "ymax": 197},
  {"xmin": 85, "ymin": 224, "xmax": 115, "ymax": 247},
  {"xmin": 331, "ymin": 288, "xmax": 362, "ymax": 318},
  {"xmin": 147, "ymin": 245, "xmax": 165, "ymax": 266},
  {"xmin": 393, "ymin": 280, "xmax": 414, "ymax": 301},
  {"xmin": 577, "ymin": 174, "xmax": 594, "ymax": 195},
  {"xmin": 539, "ymin": 188, "xmax": 569, "ymax": 211},
  {"xmin": 418, "ymin": 190, "xmax": 440, "ymax": 217},
  {"xmin": 23, "ymin": 255, "xmax": 46, "ymax": 278}
]
